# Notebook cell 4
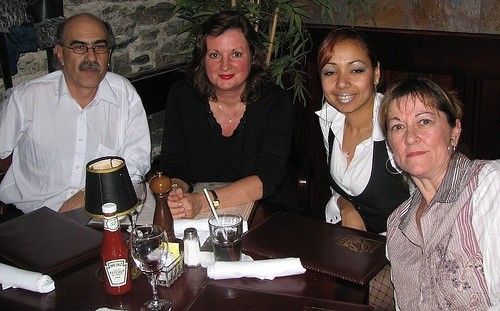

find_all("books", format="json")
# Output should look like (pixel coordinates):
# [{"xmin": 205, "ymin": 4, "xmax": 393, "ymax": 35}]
[
  {"xmin": 83, "ymin": 182, "xmax": 257, "ymax": 234},
  {"xmin": 0, "ymin": 206, "xmax": 103, "ymax": 275}
]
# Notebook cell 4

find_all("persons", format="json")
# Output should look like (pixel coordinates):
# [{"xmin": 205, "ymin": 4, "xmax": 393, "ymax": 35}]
[
  {"xmin": 160, "ymin": 9, "xmax": 293, "ymax": 219},
  {"xmin": 292, "ymin": 26, "xmax": 416, "ymax": 311},
  {"xmin": 0, "ymin": 12, "xmax": 152, "ymax": 223},
  {"xmin": 377, "ymin": 71, "xmax": 500, "ymax": 311}
]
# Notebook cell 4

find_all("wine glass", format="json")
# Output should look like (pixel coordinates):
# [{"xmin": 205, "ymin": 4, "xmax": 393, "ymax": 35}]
[
  {"xmin": 128, "ymin": 173, "xmax": 147, "ymax": 231},
  {"xmin": 130, "ymin": 224, "xmax": 172, "ymax": 311}
]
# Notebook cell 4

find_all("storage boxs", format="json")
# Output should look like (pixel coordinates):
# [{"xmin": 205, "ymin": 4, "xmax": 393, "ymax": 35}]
[{"xmin": 148, "ymin": 241, "xmax": 185, "ymax": 288}]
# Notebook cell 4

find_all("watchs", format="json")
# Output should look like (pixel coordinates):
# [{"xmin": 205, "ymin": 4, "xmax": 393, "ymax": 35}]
[{"xmin": 209, "ymin": 189, "xmax": 220, "ymax": 210}]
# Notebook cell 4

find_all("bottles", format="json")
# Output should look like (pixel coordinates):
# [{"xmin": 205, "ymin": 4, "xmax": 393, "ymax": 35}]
[
  {"xmin": 101, "ymin": 203, "xmax": 132, "ymax": 295},
  {"xmin": 149, "ymin": 171, "xmax": 176, "ymax": 242},
  {"xmin": 183, "ymin": 228, "xmax": 201, "ymax": 268}
]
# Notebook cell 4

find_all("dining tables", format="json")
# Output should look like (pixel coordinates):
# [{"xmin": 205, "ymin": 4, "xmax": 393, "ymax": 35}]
[{"xmin": 0, "ymin": 204, "xmax": 375, "ymax": 311}]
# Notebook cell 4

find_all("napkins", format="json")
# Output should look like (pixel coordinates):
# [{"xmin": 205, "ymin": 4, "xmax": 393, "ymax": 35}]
[
  {"xmin": 0, "ymin": 263, "xmax": 56, "ymax": 294},
  {"xmin": 173, "ymin": 218, "xmax": 249, "ymax": 248},
  {"xmin": 200, "ymin": 251, "xmax": 255, "ymax": 269},
  {"xmin": 206, "ymin": 255, "xmax": 308, "ymax": 282}
]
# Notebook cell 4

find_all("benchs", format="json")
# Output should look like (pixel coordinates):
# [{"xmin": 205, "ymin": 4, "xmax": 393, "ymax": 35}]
[
  {"xmin": 262, "ymin": 18, "xmax": 500, "ymax": 161},
  {"xmin": 125, "ymin": 60, "xmax": 190, "ymax": 116}
]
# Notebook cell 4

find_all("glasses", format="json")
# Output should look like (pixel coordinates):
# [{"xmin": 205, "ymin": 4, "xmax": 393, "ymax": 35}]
[{"xmin": 60, "ymin": 42, "xmax": 109, "ymax": 55}]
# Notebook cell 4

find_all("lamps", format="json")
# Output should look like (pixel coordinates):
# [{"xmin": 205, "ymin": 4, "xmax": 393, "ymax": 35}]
[{"xmin": 82, "ymin": 156, "xmax": 142, "ymax": 283}]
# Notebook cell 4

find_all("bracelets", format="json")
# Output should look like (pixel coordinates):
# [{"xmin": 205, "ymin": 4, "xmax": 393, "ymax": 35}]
[{"xmin": 171, "ymin": 183, "xmax": 180, "ymax": 187}]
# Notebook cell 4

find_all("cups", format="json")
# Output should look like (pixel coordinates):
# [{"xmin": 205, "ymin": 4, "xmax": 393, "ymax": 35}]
[
  {"xmin": 154, "ymin": 251, "xmax": 185, "ymax": 289},
  {"xmin": 208, "ymin": 214, "xmax": 243, "ymax": 261}
]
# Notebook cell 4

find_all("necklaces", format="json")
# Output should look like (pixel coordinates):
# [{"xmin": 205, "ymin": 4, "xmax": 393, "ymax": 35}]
[
  {"xmin": 345, "ymin": 121, "xmax": 371, "ymax": 157},
  {"xmin": 219, "ymin": 105, "xmax": 232, "ymax": 123}
]
